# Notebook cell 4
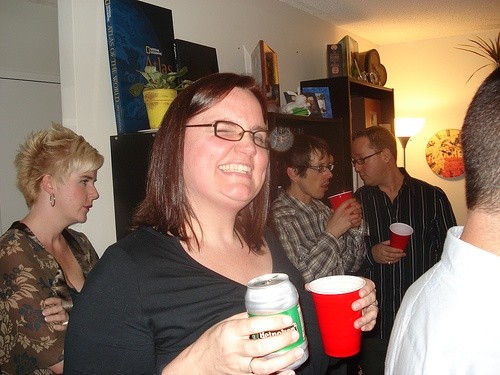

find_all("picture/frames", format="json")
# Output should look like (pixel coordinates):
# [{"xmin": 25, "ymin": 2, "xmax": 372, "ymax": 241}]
[
  {"xmin": 260, "ymin": 40, "xmax": 281, "ymax": 108},
  {"xmin": 284, "ymin": 92, "xmax": 322, "ymax": 118},
  {"xmin": 302, "ymin": 86, "xmax": 334, "ymax": 119}
]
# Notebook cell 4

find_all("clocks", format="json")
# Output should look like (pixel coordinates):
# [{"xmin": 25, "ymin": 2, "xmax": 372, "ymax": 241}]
[{"xmin": 425, "ymin": 128, "xmax": 466, "ymax": 179}]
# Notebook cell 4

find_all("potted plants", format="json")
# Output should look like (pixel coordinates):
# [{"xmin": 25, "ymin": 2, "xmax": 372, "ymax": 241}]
[{"xmin": 129, "ymin": 65, "xmax": 194, "ymax": 129}]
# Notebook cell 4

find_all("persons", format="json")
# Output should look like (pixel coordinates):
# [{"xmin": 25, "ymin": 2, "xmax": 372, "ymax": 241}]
[
  {"xmin": 0, "ymin": 122, "xmax": 104, "ymax": 375},
  {"xmin": 271, "ymin": 134, "xmax": 369, "ymax": 284},
  {"xmin": 349, "ymin": 126, "xmax": 458, "ymax": 375},
  {"xmin": 62, "ymin": 72, "xmax": 378, "ymax": 375},
  {"xmin": 385, "ymin": 65, "xmax": 500, "ymax": 375}
]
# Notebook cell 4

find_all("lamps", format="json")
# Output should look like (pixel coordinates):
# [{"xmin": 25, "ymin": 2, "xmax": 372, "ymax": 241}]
[{"xmin": 395, "ymin": 117, "xmax": 425, "ymax": 169}]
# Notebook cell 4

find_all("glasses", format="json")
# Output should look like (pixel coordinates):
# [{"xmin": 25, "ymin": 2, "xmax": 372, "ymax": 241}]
[
  {"xmin": 307, "ymin": 163, "xmax": 334, "ymax": 172},
  {"xmin": 186, "ymin": 121, "xmax": 277, "ymax": 150},
  {"xmin": 351, "ymin": 151, "xmax": 383, "ymax": 166}
]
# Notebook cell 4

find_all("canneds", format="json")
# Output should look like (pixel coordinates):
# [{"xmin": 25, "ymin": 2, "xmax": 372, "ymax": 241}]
[{"xmin": 245, "ymin": 273, "xmax": 310, "ymax": 374}]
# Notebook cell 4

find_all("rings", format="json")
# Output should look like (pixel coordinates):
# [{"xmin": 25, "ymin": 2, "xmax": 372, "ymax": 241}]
[
  {"xmin": 387, "ymin": 261, "xmax": 391, "ymax": 264},
  {"xmin": 248, "ymin": 357, "xmax": 258, "ymax": 375}
]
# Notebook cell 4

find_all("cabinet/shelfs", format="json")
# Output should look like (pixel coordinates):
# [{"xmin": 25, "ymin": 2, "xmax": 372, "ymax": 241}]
[
  {"xmin": 110, "ymin": 132, "xmax": 157, "ymax": 241},
  {"xmin": 267, "ymin": 112, "xmax": 349, "ymax": 242},
  {"xmin": 299, "ymin": 75, "xmax": 396, "ymax": 193}
]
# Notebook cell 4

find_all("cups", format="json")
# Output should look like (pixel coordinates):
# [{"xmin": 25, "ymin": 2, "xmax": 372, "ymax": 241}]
[
  {"xmin": 307, "ymin": 275, "xmax": 366, "ymax": 357},
  {"xmin": 328, "ymin": 191, "xmax": 354, "ymax": 215},
  {"xmin": 390, "ymin": 223, "xmax": 413, "ymax": 253}
]
